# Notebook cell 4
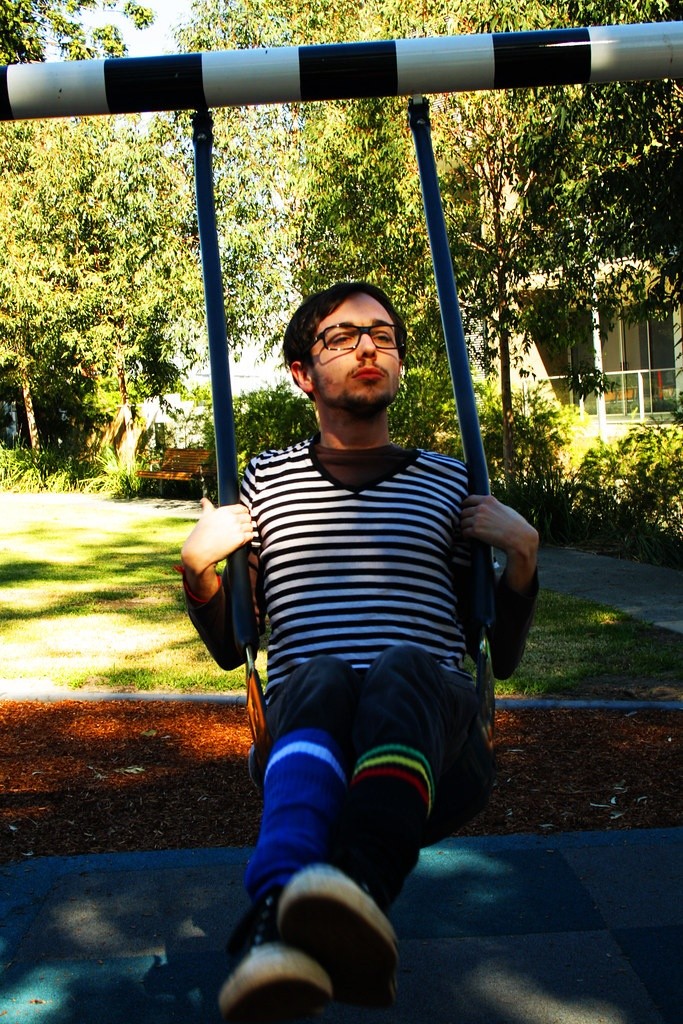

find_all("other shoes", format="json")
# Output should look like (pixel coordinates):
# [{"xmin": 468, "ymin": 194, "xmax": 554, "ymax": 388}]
[
  {"xmin": 217, "ymin": 941, "xmax": 334, "ymax": 1024},
  {"xmin": 275, "ymin": 864, "xmax": 402, "ymax": 1009}
]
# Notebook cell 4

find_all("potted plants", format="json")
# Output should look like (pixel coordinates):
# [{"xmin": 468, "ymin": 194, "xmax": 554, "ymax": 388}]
[{"xmin": 603, "ymin": 373, "xmax": 638, "ymax": 401}]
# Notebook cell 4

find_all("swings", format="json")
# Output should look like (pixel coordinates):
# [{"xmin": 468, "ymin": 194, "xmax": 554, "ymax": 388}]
[{"xmin": 185, "ymin": 91, "xmax": 511, "ymax": 856}]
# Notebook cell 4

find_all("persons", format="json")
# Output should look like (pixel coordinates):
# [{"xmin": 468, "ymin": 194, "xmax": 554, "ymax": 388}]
[{"xmin": 179, "ymin": 278, "xmax": 540, "ymax": 1021}]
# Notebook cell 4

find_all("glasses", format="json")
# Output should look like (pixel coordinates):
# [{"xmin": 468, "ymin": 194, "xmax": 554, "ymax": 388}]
[{"xmin": 303, "ymin": 324, "xmax": 407, "ymax": 354}]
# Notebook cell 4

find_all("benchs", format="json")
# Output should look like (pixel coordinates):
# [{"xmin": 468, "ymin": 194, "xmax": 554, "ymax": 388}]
[{"xmin": 134, "ymin": 449, "xmax": 213, "ymax": 498}]
